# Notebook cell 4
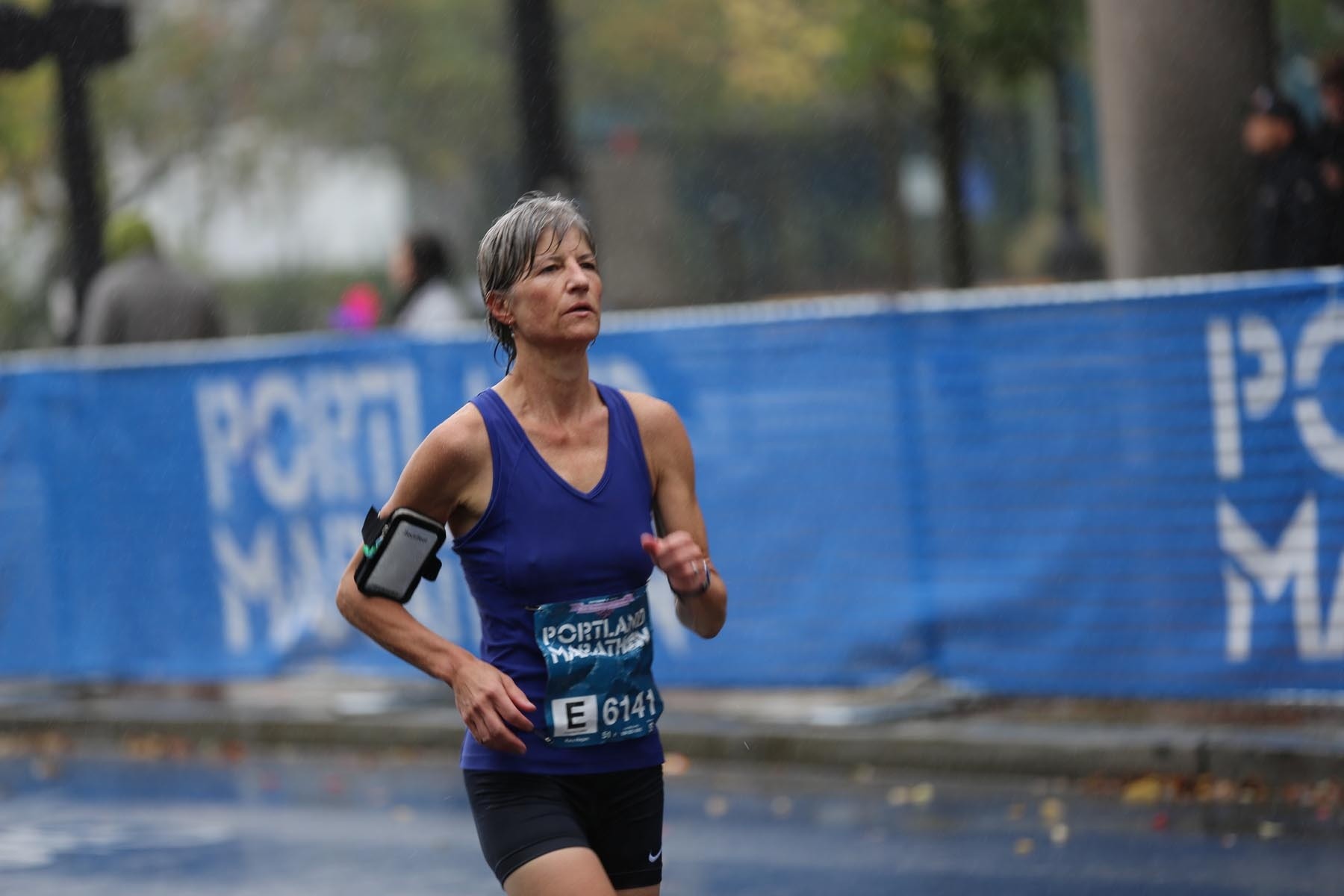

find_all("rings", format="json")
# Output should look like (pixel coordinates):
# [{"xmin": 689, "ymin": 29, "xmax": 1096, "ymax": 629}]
[{"xmin": 690, "ymin": 561, "xmax": 699, "ymax": 575}]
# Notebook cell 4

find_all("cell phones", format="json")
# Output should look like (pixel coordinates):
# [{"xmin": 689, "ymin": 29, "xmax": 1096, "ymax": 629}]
[{"xmin": 358, "ymin": 508, "xmax": 447, "ymax": 606}]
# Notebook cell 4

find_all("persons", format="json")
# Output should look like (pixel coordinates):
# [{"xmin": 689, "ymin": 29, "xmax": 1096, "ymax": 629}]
[
  {"xmin": 390, "ymin": 224, "xmax": 463, "ymax": 332},
  {"xmin": 1224, "ymin": 53, "xmax": 1344, "ymax": 271},
  {"xmin": 336, "ymin": 196, "xmax": 727, "ymax": 896},
  {"xmin": 79, "ymin": 204, "xmax": 229, "ymax": 346}
]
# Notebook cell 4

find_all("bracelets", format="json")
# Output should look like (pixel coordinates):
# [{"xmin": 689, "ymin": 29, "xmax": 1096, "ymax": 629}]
[{"xmin": 667, "ymin": 560, "xmax": 711, "ymax": 598}]
